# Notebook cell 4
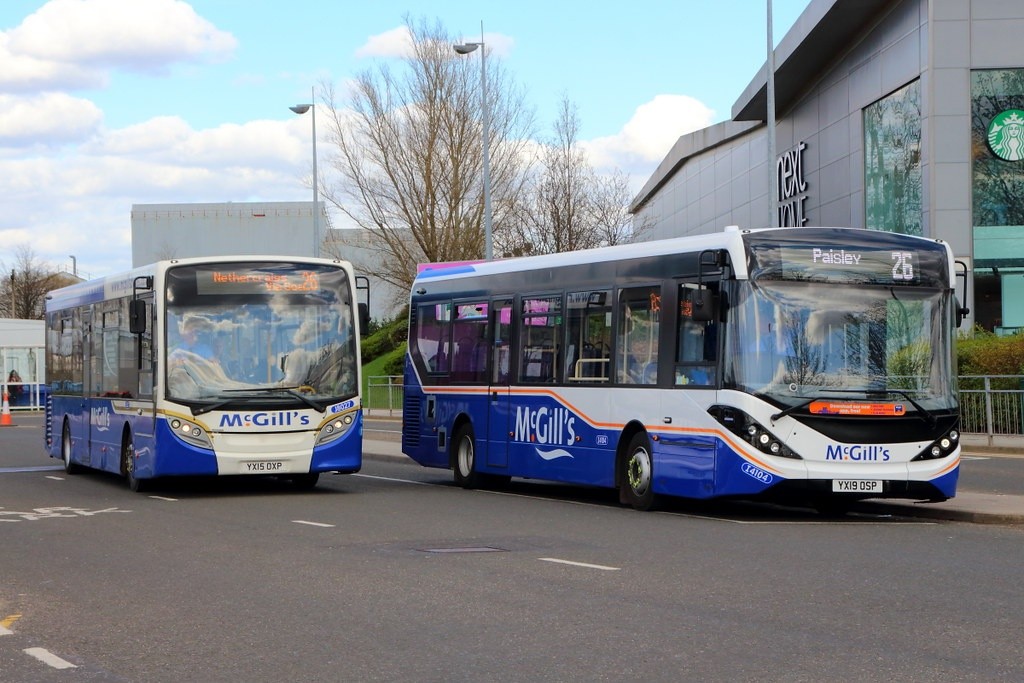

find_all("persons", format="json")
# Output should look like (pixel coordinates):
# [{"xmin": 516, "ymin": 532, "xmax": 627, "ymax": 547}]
[
  {"xmin": 172, "ymin": 321, "xmax": 216, "ymax": 362},
  {"xmin": 8, "ymin": 370, "xmax": 23, "ymax": 405}
]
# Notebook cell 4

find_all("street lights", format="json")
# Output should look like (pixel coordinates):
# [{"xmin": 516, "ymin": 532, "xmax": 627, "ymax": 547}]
[
  {"xmin": 289, "ymin": 104, "xmax": 319, "ymax": 259},
  {"xmin": 453, "ymin": 42, "xmax": 494, "ymax": 261}
]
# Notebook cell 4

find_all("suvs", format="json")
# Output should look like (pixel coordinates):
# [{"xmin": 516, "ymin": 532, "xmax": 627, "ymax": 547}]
[{"xmin": 44, "ymin": 254, "xmax": 371, "ymax": 491}]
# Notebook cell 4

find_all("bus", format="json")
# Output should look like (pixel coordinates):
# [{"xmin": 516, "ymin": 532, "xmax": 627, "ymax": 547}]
[{"xmin": 401, "ymin": 225, "xmax": 969, "ymax": 511}]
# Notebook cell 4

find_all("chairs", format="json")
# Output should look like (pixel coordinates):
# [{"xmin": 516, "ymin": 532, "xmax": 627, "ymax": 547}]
[{"xmin": 428, "ymin": 338, "xmax": 613, "ymax": 385}]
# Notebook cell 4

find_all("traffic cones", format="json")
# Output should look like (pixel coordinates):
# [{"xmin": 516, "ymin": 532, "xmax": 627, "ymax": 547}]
[{"xmin": 0, "ymin": 394, "xmax": 18, "ymax": 426}]
